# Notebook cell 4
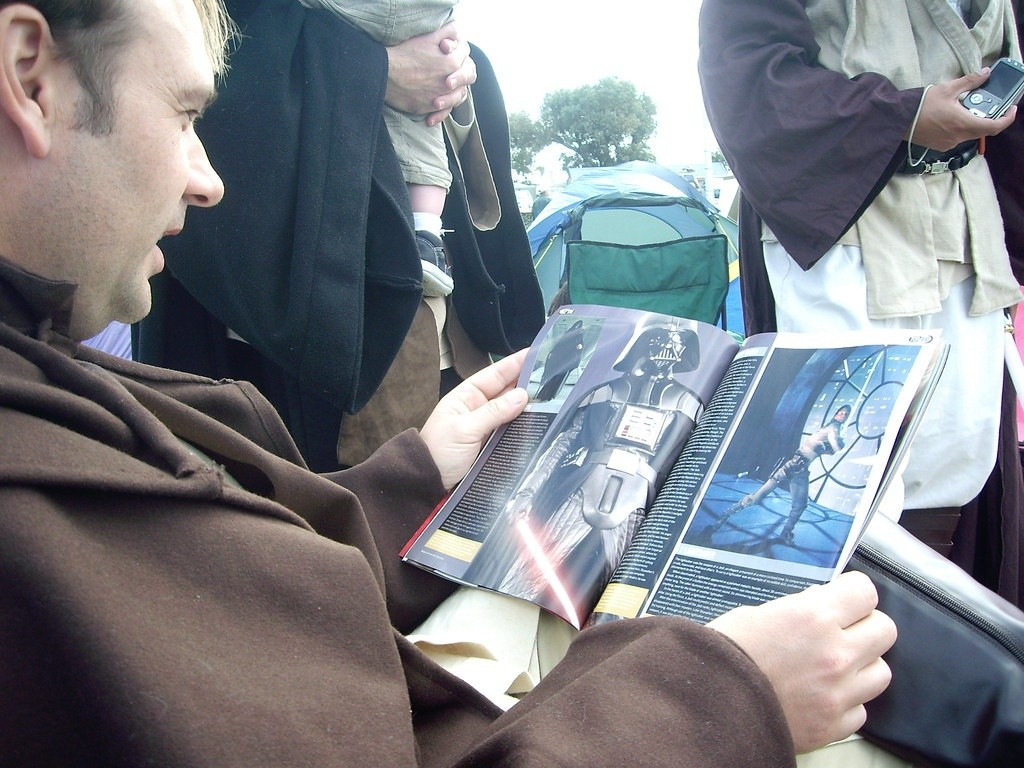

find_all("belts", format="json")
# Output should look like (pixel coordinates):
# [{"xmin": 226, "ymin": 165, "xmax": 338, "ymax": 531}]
[
  {"xmin": 899, "ymin": 142, "xmax": 983, "ymax": 175},
  {"xmin": 585, "ymin": 450, "xmax": 656, "ymax": 484}
]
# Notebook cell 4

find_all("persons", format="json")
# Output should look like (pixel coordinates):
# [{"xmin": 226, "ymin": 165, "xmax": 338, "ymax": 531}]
[
  {"xmin": 1, "ymin": 1, "xmax": 1022, "ymax": 768},
  {"xmin": 702, "ymin": 405, "xmax": 852, "ymax": 545}
]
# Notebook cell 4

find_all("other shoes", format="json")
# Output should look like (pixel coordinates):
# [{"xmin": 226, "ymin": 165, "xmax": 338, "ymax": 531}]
[
  {"xmin": 781, "ymin": 530, "xmax": 795, "ymax": 546},
  {"xmin": 713, "ymin": 513, "xmax": 727, "ymax": 533},
  {"xmin": 416, "ymin": 228, "xmax": 454, "ymax": 297}
]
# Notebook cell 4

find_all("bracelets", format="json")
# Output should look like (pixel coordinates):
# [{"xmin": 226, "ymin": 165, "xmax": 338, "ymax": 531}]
[{"xmin": 907, "ymin": 75, "xmax": 933, "ymax": 167}]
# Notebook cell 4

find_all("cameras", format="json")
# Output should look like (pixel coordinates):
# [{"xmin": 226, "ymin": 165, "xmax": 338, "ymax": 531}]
[{"xmin": 963, "ymin": 57, "xmax": 1024, "ymax": 120}]
